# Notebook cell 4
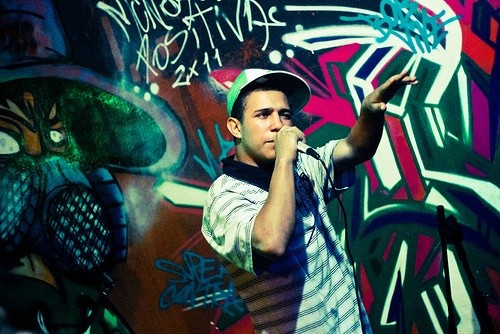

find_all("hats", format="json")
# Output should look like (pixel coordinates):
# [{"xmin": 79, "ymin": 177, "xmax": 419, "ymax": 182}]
[{"xmin": 227, "ymin": 68, "xmax": 312, "ymax": 118}]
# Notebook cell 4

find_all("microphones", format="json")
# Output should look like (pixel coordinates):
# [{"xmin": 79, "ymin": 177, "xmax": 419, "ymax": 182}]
[{"xmin": 297, "ymin": 140, "xmax": 320, "ymax": 160}]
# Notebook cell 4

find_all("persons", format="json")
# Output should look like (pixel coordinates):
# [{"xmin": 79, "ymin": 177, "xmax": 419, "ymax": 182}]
[{"xmin": 201, "ymin": 68, "xmax": 419, "ymax": 334}]
